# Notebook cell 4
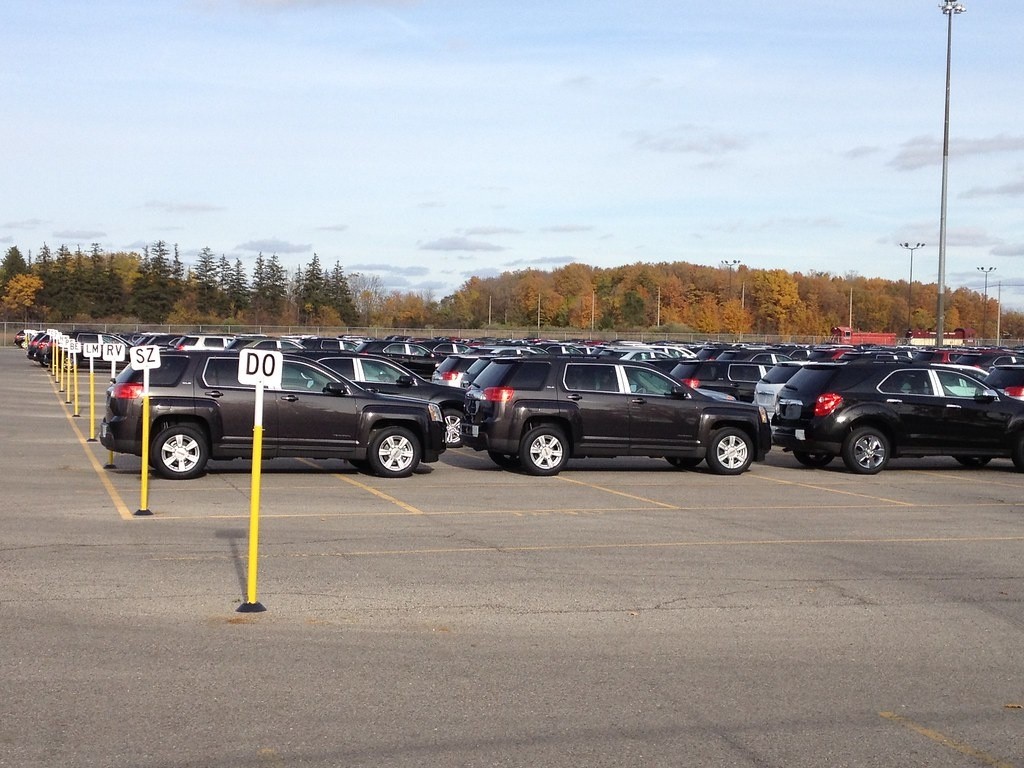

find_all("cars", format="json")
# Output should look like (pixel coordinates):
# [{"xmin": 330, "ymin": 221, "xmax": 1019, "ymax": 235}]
[
  {"xmin": 769, "ymin": 358, "xmax": 1024, "ymax": 475},
  {"xmin": 461, "ymin": 357, "xmax": 772, "ymax": 478},
  {"xmin": 976, "ymin": 355, "xmax": 1024, "ymax": 402},
  {"xmin": 14, "ymin": 328, "xmax": 1024, "ymax": 392},
  {"xmin": 291, "ymin": 348, "xmax": 470, "ymax": 449},
  {"xmin": 99, "ymin": 350, "xmax": 448, "ymax": 478}
]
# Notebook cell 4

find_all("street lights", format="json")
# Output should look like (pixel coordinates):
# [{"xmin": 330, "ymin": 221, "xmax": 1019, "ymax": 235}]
[
  {"xmin": 899, "ymin": 242, "xmax": 926, "ymax": 329},
  {"xmin": 976, "ymin": 266, "xmax": 997, "ymax": 346},
  {"xmin": 721, "ymin": 260, "xmax": 740, "ymax": 295},
  {"xmin": 934, "ymin": 1, "xmax": 967, "ymax": 346}
]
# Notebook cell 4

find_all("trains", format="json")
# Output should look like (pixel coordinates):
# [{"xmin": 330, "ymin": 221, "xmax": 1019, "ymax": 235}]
[{"xmin": 829, "ymin": 327, "xmax": 973, "ymax": 350}]
